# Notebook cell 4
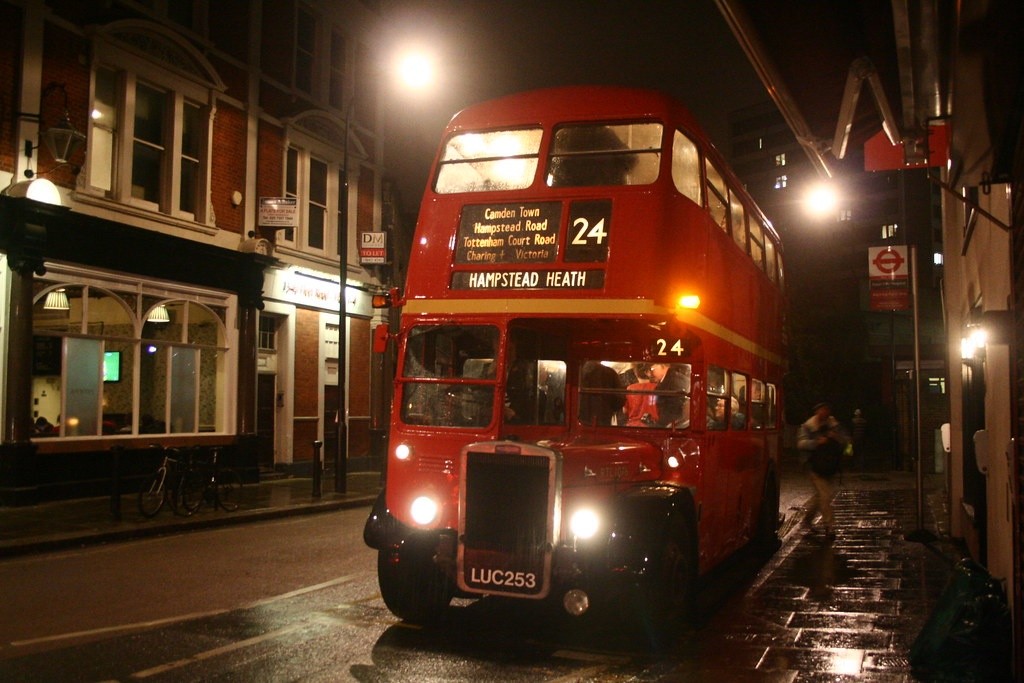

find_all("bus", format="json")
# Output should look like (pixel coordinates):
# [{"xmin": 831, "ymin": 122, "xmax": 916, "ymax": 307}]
[{"xmin": 360, "ymin": 87, "xmax": 786, "ymax": 649}]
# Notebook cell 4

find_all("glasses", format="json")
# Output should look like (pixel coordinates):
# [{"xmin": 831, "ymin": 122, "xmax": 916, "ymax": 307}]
[{"xmin": 646, "ymin": 368, "xmax": 655, "ymax": 373}]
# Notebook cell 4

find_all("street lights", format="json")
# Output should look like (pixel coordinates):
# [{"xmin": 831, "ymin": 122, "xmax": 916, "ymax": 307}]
[{"xmin": 331, "ymin": 48, "xmax": 444, "ymax": 496}]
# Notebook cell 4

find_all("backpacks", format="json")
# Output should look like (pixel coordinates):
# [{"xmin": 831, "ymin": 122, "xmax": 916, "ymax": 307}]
[{"xmin": 808, "ymin": 425, "xmax": 844, "ymax": 476}]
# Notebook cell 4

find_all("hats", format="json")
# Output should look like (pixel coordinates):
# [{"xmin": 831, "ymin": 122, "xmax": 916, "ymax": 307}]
[{"xmin": 812, "ymin": 398, "xmax": 828, "ymax": 410}]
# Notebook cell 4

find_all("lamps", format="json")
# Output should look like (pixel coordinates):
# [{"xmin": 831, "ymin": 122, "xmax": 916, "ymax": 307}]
[
  {"xmin": 42, "ymin": 292, "xmax": 69, "ymax": 310},
  {"xmin": 38, "ymin": 81, "xmax": 87, "ymax": 163},
  {"xmin": 147, "ymin": 306, "xmax": 169, "ymax": 322}
]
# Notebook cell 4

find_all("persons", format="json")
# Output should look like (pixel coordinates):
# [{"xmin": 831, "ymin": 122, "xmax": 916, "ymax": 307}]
[
  {"xmin": 140, "ymin": 414, "xmax": 156, "ymax": 434},
  {"xmin": 796, "ymin": 402, "xmax": 848, "ymax": 540},
  {"xmin": 121, "ymin": 413, "xmax": 132, "ymax": 432},
  {"xmin": 504, "ymin": 355, "xmax": 779, "ymax": 427},
  {"xmin": 35, "ymin": 415, "xmax": 63, "ymax": 436}
]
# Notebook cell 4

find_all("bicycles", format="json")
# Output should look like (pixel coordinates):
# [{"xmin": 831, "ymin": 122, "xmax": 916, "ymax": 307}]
[{"xmin": 134, "ymin": 441, "xmax": 246, "ymax": 517}]
